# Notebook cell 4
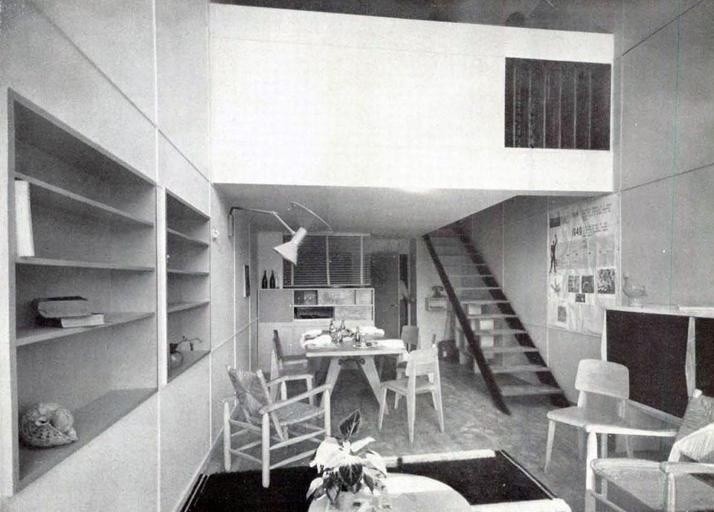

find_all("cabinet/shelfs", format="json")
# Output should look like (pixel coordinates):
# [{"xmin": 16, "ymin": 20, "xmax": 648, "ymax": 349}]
[
  {"xmin": 453, "ymin": 304, "xmax": 494, "ymax": 374},
  {"xmin": 6, "ymin": 88, "xmax": 162, "ymax": 512},
  {"xmin": 257, "ymin": 287, "xmax": 374, "ymax": 374},
  {"xmin": 160, "ymin": 184, "xmax": 210, "ymax": 503}
]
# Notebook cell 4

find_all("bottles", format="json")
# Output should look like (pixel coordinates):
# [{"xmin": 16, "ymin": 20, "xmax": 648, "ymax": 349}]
[
  {"xmin": 260, "ymin": 270, "xmax": 278, "ymax": 288},
  {"xmin": 329, "ymin": 319, "xmax": 367, "ymax": 348},
  {"xmin": 169, "ymin": 342, "xmax": 184, "ymax": 368}
]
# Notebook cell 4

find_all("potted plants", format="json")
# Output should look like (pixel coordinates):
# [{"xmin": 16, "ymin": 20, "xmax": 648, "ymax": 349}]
[{"xmin": 305, "ymin": 407, "xmax": 388, "ymax": 504}]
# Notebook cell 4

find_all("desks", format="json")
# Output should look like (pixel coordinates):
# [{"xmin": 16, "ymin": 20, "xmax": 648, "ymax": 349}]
[{"xmin": 303, "ymin": 332, "xmax": 403, "ymax": 418}]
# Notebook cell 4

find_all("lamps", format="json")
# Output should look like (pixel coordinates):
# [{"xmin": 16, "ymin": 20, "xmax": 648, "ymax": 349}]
[{"xmin": 227, "ymin": 205, "xmax": 307, "ymax": 266}]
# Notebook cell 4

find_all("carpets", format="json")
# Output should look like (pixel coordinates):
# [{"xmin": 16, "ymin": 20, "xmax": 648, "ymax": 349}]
[{"xmin": 182, "ymin": 448, "xmax": 572, "ymax": 512}]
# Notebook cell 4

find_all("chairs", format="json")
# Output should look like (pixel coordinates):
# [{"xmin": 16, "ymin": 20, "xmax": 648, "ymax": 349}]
[
  {"xmin": 221, "ymin": 328, "xmax": 332, "ymax": 488},
  {"xmin": 377, "ymin": 325, "xmax": 444, "ymax": 443},
  {"xmin": 542, "ymin": 358, "xmax": 714, "ymax": 512}
]
